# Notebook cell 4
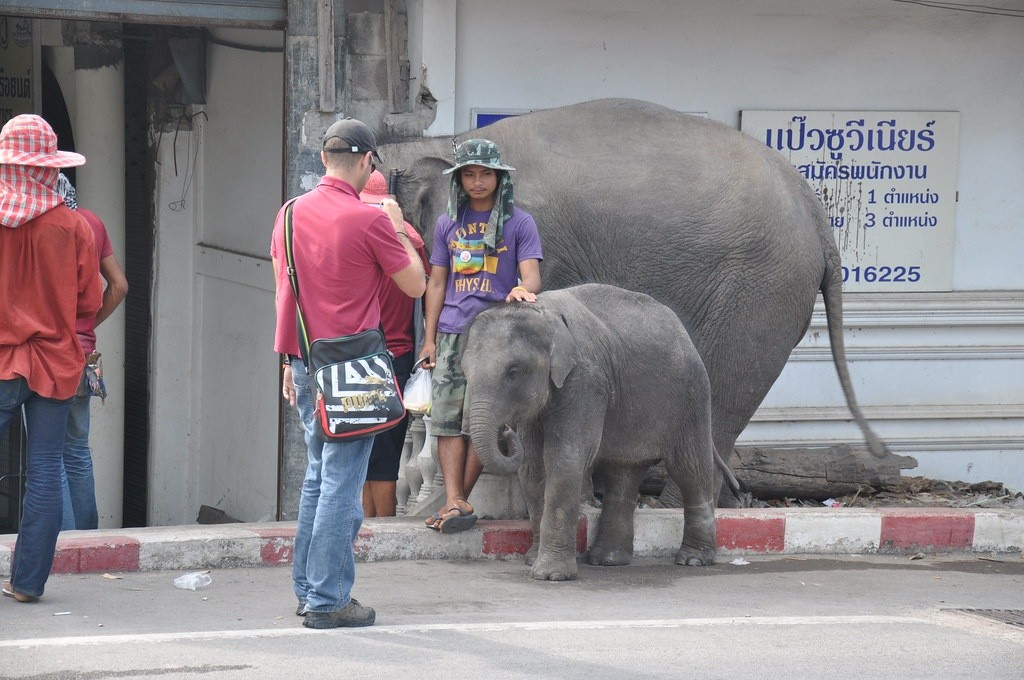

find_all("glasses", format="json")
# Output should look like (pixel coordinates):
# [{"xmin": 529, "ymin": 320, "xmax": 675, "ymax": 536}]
[{"xmin": 370, "ymin": 155, "xmax": 376, "ymax": 173}]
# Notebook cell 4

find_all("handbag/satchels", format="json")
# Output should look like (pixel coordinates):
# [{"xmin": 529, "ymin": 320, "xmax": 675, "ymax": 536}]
[
  {"xmin": 403, "ymin": 363, "xmax": 433, "ymax": 417},
  {"xmin": 455, "ymin": 237, "xmax": 485, "ymax": 275},
  {"xmin": 305, "ymin": 329, "xmax": 406, "ymax": 443}
]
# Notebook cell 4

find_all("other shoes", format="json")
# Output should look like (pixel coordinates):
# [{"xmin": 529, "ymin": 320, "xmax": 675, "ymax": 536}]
[{"xmin": 2, "ymin": 588, "xmax": 39, "ymax": 602}]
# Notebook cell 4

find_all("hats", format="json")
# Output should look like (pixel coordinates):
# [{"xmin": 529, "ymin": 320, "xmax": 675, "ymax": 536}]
[
  {"xmin": 442, "ymin": 139, "xmax": 516, "ymax": 175},
  {"xmin": 322, "ymin": 117, "xmax": 385, "ymax": 164},
  {"xmin": 359, "ymin": 170, "xmax": 396, "ymax": 204},
  {"xmin": 0, "ymin": 114, "xmax": 86, "ymax": 168}
]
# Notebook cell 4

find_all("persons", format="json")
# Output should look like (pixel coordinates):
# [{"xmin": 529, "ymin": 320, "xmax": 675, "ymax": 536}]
[
  {"xmin": 0, "ymin": 112, "xmax": 128, "ymax": 604},
  {"xmin": 270, "ymin": 118, "xmax": 425, "ymax": 630},
  {"xmin": 358, "ymin": 170, "xmax": 424, "ymax": 518},
  {"xmin": 420, "ymin": 139, "xmax": 544, "ymax": 531}
]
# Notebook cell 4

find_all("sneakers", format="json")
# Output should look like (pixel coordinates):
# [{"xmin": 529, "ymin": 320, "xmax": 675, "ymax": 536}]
[{"xmin": 297, "ymin": 597, "xmax": 375, "ymax": 629}]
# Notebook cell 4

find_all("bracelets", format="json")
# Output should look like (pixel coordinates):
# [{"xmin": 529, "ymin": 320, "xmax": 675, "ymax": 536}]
[
  {"xmin": 282, "ymin": 360, "xmax": 290, "ymax": 369},
  {"xmin": 512, "ymin": 286, "xmax": 527, "ymax": 292},
  {"xmin": 395, "ymin": 231, "xmax": 408, "ymax": 238}
]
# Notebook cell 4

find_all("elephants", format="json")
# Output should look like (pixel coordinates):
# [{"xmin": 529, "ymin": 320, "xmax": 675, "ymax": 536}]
[
  {"xmin": 359, "ymin": 99, "xmax": 891, "ymax": 508},
  {"xmin": 461, "ymin": 284, "xmax": 716, "ymax": 581}
]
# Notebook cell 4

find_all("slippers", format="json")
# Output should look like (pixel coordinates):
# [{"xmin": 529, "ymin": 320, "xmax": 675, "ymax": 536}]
[{"xmin": 426, "ymin": 507, "xmax": 478, "ymax": 533}]
